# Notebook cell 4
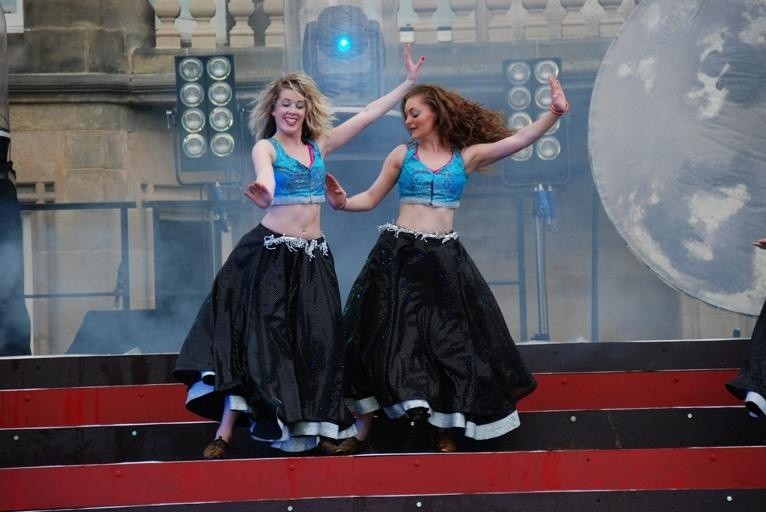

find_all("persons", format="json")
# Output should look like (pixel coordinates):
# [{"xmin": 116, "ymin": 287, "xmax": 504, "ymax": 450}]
[
  {"xmin": 721, "ymin": 235, "xmax": 766, "ymax": 420},
  {"xmin": 171, "ymin": 40, "xmax": 428, "ymax": 459},
  {"xmin": 321, "ymin": 69, "xmax": 571, "ymax": 452}
]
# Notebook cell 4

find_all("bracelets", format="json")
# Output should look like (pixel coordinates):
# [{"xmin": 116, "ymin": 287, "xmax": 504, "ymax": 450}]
[
  {"xmin": 337, "ymin": 199, "xmax": 346, "ymax": 210},
  {"xmin": 549, "ymin": 101, "xmax": 570, "ymax": 117}
]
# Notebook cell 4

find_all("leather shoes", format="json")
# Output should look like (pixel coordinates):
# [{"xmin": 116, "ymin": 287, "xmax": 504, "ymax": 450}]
[
  {"xmin": 434, "ymin": 428, "xmax": 456, "ymax": 452},
  {"xmin": 315, "ymin": 437, "xmax": 339, "ymax": 453},
  {"xmin": 335, "ymin": 435, "xmax": 369, "ymax": 455},
  {"xmin": 203, "ymin": 434, "xmax": 230, "ymax": 459}
]
